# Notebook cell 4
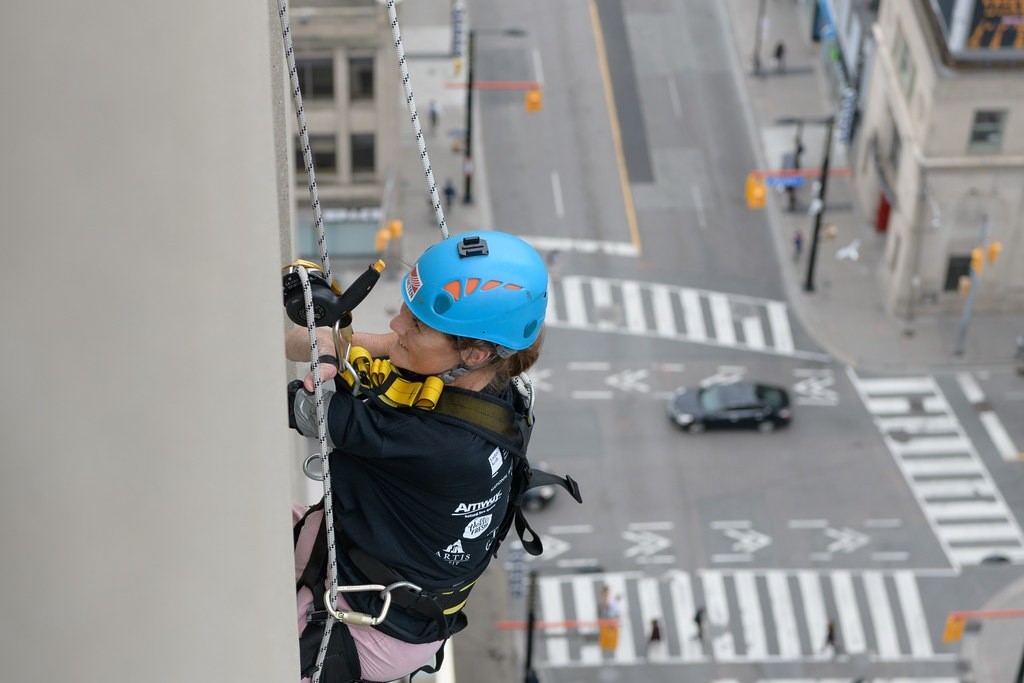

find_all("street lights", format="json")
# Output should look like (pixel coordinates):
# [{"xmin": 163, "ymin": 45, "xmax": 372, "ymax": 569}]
[
  {"xmin": 776, "ymin": 114, "xmax": 837, "ymax": 294},
  {"xmin": 463, "ymin": 28, "xmax": 530, "ymax": 203}
]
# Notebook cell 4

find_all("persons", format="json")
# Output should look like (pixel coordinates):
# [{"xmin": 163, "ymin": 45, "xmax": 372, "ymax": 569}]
[
  {"xmin": 286, "ymin": 228, "xmax": 550, "ymax": 683},
  {"xmin": 444, "ymin": 183, "xmax": 455, "ymax": 206},
  {"xmin": 695, "ymin": 608, "xmax": 706, "ymax": 638},
  {"xmin": 824, "ymin": 623, "xmax": 834, "ymax": 645},
  {"xmin": 651, "ymin": 620, "xmax": 660, "ymax": 640},
  {"xmin": 429, "ymin": 101, "xmax": 439, "ymax": 124},
  {"xmin": 793, "ymin": 233, "xmax": 803, "ymax": 261},
  {"xmin": 599, "ymin": 587, "xmax": 620, "ymax": 617},
  {"xmin": 775, "ymin": 44, "xmax": 784, "ymax": 62}
]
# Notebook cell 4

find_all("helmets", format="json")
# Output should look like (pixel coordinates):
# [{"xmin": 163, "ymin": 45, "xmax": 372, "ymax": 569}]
[{"xmin": 400, "ymin": 230, "xmax": 548, "ymax": 351}]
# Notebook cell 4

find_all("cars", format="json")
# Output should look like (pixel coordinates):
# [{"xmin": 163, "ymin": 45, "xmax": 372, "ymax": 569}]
[
  {"xmin": 524, "ymin": 461, "xmax": 557, "ymax": 512},
  {"xmin": 668, "ymin": 379, "xmax": 793, "ymax": 437}
]
